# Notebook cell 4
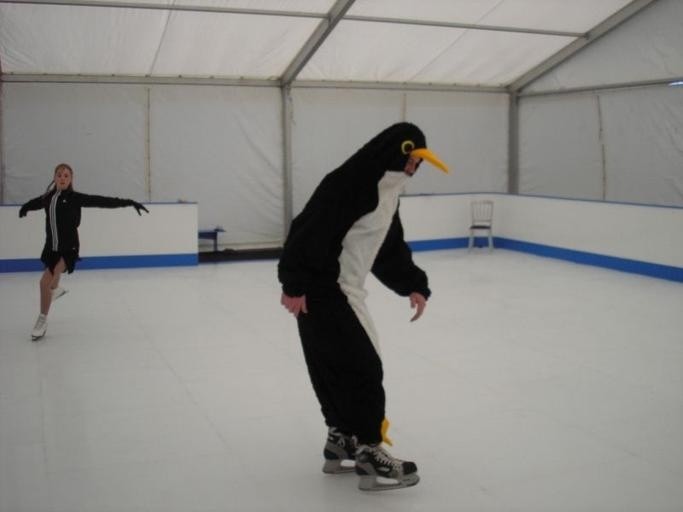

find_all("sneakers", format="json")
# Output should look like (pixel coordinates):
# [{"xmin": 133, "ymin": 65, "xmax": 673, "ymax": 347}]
[
  {"xmin": 50, "ymin": 285, "xmax": 65, "ymax": 302},
  {"xmin": 31, "ymin": 311, "xmax": 49, "ymax": 338},
  {"xmin": 323, "ymin": 428, "xmax": 357, "ymax": 462},
  {"xmin": 353, "ymin": 444, "xmax": 417, "ymax": 478}
]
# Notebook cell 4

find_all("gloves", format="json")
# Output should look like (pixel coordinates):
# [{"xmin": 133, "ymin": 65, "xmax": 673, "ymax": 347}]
[
  {"xmin": 18, "ymin": 203, "xmax": 31, "ymax": 218},
  {"xmin": 125, "ymin": 199, "xmax": 150, "ymax": 215}
]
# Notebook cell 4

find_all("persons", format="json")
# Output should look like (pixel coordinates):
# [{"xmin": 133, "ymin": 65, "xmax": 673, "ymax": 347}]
[
  {"xmin": 18, "ymin": 163, "xmax": 148, "ymax": 342},
  {"xmin": 277, "ymin": 120, "xmax": 448, "ymax": 494}
]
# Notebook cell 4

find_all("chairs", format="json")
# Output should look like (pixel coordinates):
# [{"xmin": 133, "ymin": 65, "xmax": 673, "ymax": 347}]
[{"xmin": 466, "ymin": 200, "xmax": 494, "ymax": 253}]
